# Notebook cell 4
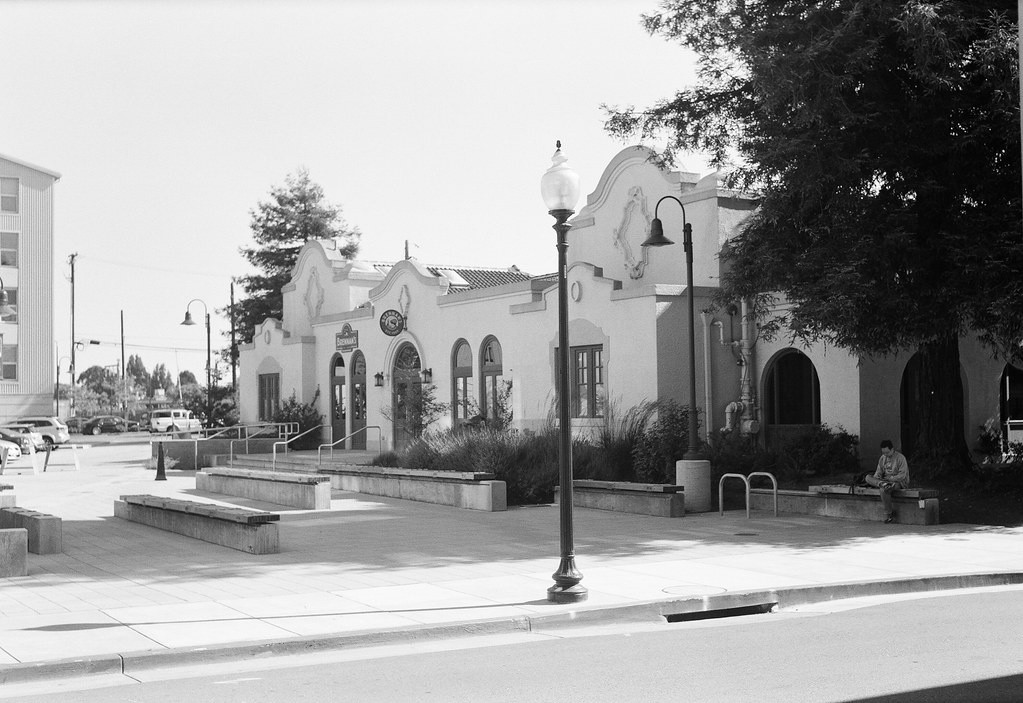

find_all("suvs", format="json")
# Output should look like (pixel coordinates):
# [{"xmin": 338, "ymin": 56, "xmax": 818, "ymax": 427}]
[{"xmin": 0, "ymin": 415, "xmax": 71, "ymax": 451}]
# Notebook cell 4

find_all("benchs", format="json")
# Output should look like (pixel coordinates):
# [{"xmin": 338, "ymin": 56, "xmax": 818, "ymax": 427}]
[
  {"xmin": 196, "ymin": 467, "xmax": 332, "ymax": 510},
  {"xmin": 553, "ymin": 480, "xmax": 686, "ymax": 518},
  {"xmin": 320, "ymin": 464, "xmax": 507, "ymax": 513},
  {"xmin": 810, "ymin": 484, "xmax": 940, "ymax": 525},
  {"xmin": 114, "ymin": 493, "xmax": 282, "ymax": 555}
]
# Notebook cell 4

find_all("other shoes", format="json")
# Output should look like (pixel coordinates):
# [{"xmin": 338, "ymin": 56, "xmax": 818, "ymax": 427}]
[
  {"xmin": 884, "ymin": 482, "xmax": 897, "ymax": 494},
  {"xmin": 885, "ymin": 511, "xmax": 896, "ymax": 524}
]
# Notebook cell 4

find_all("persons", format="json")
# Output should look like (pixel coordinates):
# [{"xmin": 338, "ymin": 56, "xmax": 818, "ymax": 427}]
[{"xmin": 865, "ymin": 440, "xmax": 909, "ymax": 523}]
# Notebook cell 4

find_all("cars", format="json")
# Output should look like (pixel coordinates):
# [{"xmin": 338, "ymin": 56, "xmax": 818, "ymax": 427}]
[
  {"xmin": 63, "ymin": 418, "xmax": 89, "ymax": 432},
  {"xmin": 0, "ymin": 429, "xmax": 42, "ymax": 457},
  {"xmin": 0, "ymin": 438, "xmax": 21, "ymax": 465},
  {"xmin": 80, "ymin": 415, "xmax": 139, "ymax": 436}
]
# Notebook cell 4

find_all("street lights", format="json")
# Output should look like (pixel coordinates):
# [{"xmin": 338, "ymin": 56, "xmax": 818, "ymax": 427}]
[
  {"xmin": 639, "ymin": 196, "xmax": 706, "ymax": 462},
  {"xmin": 56, "ymin": 356, "xmax": 77, "ymax": 416},
  {"xmin": 180, "ymin": 298, "xmax": 212, "ymax": 432},
  {"xmin": 216, "ymin": 304, "xmax": 236, "ymax": 397},
  {"xmin": 542, "ymin": 138, "xmax": 590, "ymax": 605}
]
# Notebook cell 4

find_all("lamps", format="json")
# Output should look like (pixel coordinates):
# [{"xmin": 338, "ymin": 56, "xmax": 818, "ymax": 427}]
[
  {"xmin": 420, "ymin": 368, "xmax": 432, "ymax": 384},
  {"xmin": 374, "ymin": 371, "xmax": 383, "ymax": 387}
]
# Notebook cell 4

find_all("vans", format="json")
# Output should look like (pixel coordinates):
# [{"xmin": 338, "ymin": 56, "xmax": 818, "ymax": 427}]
[{"xmin": 148, "ymin": 408, "xmax": 223, "ymax": 434}]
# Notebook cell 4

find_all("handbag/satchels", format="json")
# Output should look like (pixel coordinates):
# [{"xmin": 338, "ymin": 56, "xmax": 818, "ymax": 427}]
[{"xmin": 849, "ymin": 471, "xmax": 875, "ymax": 496}]
